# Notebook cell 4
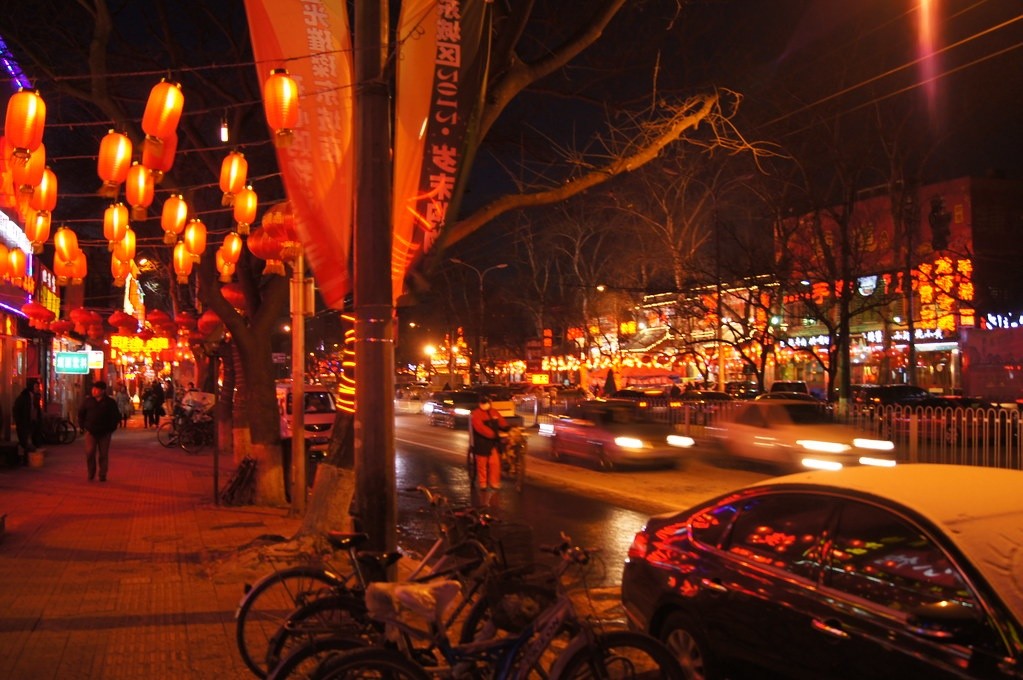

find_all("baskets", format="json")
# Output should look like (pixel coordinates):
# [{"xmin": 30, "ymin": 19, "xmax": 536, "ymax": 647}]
[{"xmin": 483, "ymin": 521, "xmax": 535, "ymax": 578}]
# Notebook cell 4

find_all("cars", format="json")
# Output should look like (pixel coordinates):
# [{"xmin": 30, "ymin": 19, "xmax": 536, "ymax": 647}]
[
  {"xmin": 676, "ymin": 380, "xmax": 732, "ymax": 425},
  {"xmin": 424, "ymin": 390, "xmax": 490, "ymax": 428},
  {"xmin": 621, "ymin": 464, "xmax": 1023, "ymax": 680},
  {"xmin": 500, "ymin": 382, "xmax": 683, "ymax": 424},
  {"xmin": 396, "ymin": 381, "xmax": 428, "ymax": 400},
  {"xmin": 848, "ymin": 383, "xmax": 1001, "ymax": 447},
  {"xmin": 552, "ymin": 400, "xmax": 681, "ymax": 470},
  {"xmin": 726, "ymin": 380, "xmax": 757, "ymax": 398},
  {"xmin": 708, "ymin": 391, "xmax": 889, "ymax": 474}
]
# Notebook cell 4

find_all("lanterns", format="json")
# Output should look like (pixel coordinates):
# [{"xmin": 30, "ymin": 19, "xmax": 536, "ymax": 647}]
[
  {"xmin": 5, "ymin": 87, "xmax": 45, "ymax": 166},
  {"xmin": 142, "ymin": 78, "xmax": 184, "ymax": 144},
  {"xmin": 0, "ymin": 129, "xmax": 305, "ymax": 366},
  {"xmin": 265, "ymin": 68, "xmax": 299, "ymax": 135}
]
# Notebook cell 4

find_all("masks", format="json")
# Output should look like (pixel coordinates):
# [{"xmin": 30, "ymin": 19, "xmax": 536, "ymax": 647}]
[{"xmin": 479, "ymin": 402, "xmax": 490, "ymax": 412}]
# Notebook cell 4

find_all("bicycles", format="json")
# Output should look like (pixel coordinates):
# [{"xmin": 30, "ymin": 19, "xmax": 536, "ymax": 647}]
[
  {"xmin": 158, "ymin": 393, "xmax": 216, "ymax": 453},
  {"xmin": 235, "ymin": 478, "xmax": 687, "ymax": 680},
  {"xmin": 467, "ymin": 430, "xmax": 527, "ymax": 492},
  {"xmin": 34, "ymin": 414, "xmax": 77, "ymax": 444}
]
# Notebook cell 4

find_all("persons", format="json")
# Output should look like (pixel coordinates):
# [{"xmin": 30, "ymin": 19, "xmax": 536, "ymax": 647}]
[
  {"xmin": 78, "ymin": 382, "xmax": 120, "ymax": 481},
  {"xmin": 671, "ymin": 382, "xmax": 700, "ymax": 395},
  {"xmin": 142, "ymin": 380, "xmax": 194, "ymax": 428},
  {"xmin": 472, "ymin": 394, "xmax": 510, "ymax": 507},
  {"xmin": 13, "ymin": 381, "xmax": 48, "ymax": 459},
  {"xmin": 116, "ymin": 386, "xmax": 130, "ymax": 427}
]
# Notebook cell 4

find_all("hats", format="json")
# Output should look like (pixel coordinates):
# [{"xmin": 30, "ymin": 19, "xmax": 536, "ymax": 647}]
[{"xmin": 92, "ymin": 382, "xmax": 106, "ymax": 391}]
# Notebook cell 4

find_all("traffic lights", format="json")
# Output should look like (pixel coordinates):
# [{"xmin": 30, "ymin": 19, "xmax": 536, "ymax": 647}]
[{"xmin": 930, "ymin": 205, "xmax": 951, "ymax": 250}]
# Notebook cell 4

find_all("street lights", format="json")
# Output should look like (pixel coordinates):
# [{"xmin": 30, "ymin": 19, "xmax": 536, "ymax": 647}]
[
  {"xmin": 449, "ymin": 257, "xmax": 509, "ymax": 383},
  {"xmin": 597, "ymin": 284, "xmax": 623, "ymax": 364},
  {"xmin": 410, "ymin": 322, "xmax": 431, "ymax": 377}
]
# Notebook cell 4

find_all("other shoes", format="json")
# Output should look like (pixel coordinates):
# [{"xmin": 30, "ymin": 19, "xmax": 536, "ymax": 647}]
[
  {"xmin": 491, "ymin": 482, "xmax": 501, "ymax": 489},
  {"xmin": 89, "ymin": 471, "xmax": 94, "ymax": 479},
  {"xmin": 99, "ymin": 473, "xmax": 106, "ymax": 482},
  {"xmin": 480, "ymin": 482, "xmax": 487, "ymax": 489}
]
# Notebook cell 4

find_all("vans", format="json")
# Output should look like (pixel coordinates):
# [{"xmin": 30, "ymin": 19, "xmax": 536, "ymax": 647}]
[
  {"xmin": 770, "ymin": 380, "xmax": 809, "ymax": 393},
  {"xmin": 428, "ymin": 373, "xmax": 463, "ymax": 390},
  {"xmin": 274, "ymin": 383, "xmax": 337, "ymax": 458}
]
